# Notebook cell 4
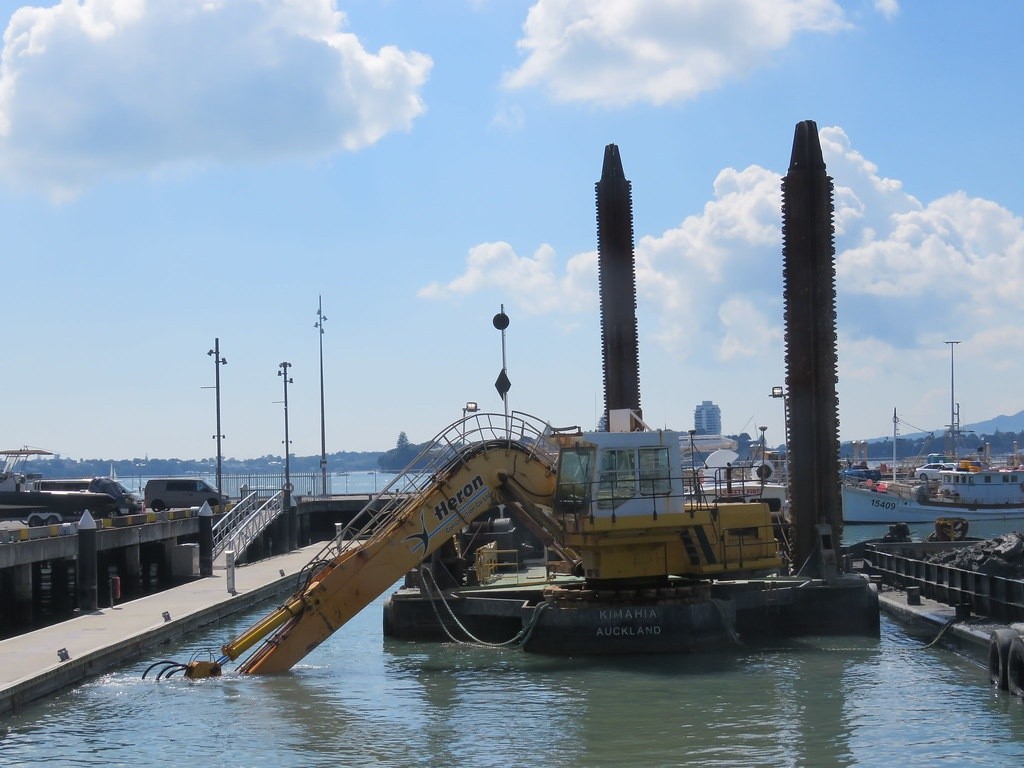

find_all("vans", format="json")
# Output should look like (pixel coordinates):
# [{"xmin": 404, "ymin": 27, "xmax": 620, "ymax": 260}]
[
  {"xmin": 144, "ymin": 479, "xmax": 231, "ymax": 512},
  {"xmin": 33, "ymin": 479, "xmax": 145, "ymax": 520}
]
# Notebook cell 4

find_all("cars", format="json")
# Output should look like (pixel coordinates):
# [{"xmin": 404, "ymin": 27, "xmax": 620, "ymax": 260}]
[{"xmin": 914, "ymin": 463, "xmax": 961, "ymax": 481}]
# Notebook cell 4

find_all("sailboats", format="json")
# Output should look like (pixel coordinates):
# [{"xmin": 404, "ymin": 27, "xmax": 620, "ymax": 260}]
[{"xmin": 841, "ymin": 403, "xmax": 1024, "ymax": 525}]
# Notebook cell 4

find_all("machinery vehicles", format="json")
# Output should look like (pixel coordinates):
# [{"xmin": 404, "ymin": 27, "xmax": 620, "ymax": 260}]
[{"xmin": 141, "ymin": 410, "xmax": 784, "ymax": 684}]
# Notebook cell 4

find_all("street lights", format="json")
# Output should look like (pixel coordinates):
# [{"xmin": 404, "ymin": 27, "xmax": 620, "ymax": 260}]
[
  {"xmin": 277, "ymin": 362, "xmax": 294, "ymax": 489},
  {"xmin": 207, "ymin": 338, "xmax": 228, "ymax": 511},
  {"xmin": 943, "ymin": 341, "xmax": 961, "ymax": 455},
  {"xmin": 314, "ymin": 294, "xmax": 328, "ymax": 493}
]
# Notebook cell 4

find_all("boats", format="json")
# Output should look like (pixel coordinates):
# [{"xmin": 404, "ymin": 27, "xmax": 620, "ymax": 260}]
[
  {"xmin": 384, "ymin": 120, "xmax": 883, "ymax": 655},
  {"xmin": 0, "ymin": 445, "xmax": 117, "ymax": 522}
]
